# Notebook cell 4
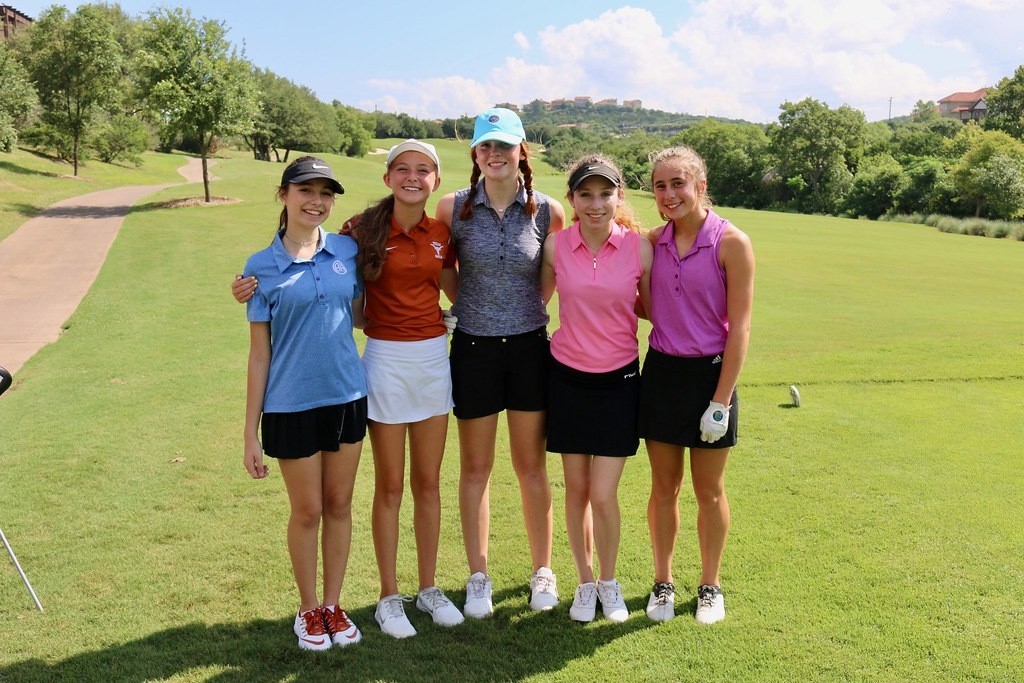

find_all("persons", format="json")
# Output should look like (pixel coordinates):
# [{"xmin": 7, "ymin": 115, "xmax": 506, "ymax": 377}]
[
  {"xmin": 242, "ymin": 156, "xmax": 365, "ymax": 651},
  {"xmin": 435, "ymin": 108, "xmax": 565, "ymax": 620},
  {"xmin": 539, "ymin": 153, "xmax": 653, "ymax": 623},
  {"xmin": 230, "ymin": 137, "xmax": 466, "ymax": 639},
  {"xmin": 634, "ymin": 144, "xmax": 755, "ymax": 624}
]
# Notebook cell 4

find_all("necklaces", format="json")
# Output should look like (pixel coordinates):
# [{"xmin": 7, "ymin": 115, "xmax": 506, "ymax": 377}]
[
  {"xmin": 493, "ymin": 205, "xmax": 504, "ymax": 213},
  {"xmin": 285, "ymin": 233, "xmax": 321, "ymax": 246}
]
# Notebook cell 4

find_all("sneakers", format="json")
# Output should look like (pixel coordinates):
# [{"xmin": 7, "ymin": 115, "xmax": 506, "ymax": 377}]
[
  {"xmin": 294, "ymin": 607, "xmax": 332, "ymax": 650},
  {"xmin": 695, "ymin": 584, "xmax": 725, "ymax": 624},
  {"xmin": 646, "ymin": 579, "xmax": 682, "ymax": 622},
  {"xmin": 464, "ymin": 572, "xmax": 494, "ymax": 620},
  {"xmin": 595, "ymin": 580, "xmax": 629, "ymax": 623},
  {"xmin": 570, "ymin": 583, "xmax": 602, "ymax": 622},
  {"xmin": 416, "ymin": 588, "xmax": 466, "ymax": 627},
  {"xmin": 320, "ymin": 604, "xmax": 362, "ymax": 647},
  {"xmin": 375, "ymin": 595, "xmax": 417, "ymax": 639},
  {"xmin": 530, "ymin": 567, "xmax": 558, "ymax": 610}
]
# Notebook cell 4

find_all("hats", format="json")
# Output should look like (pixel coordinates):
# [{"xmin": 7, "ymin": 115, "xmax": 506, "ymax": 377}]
[
  {"xmin": 569, "ymin": 162, "xmax": 622, "ymax": 195},
  {"xmin": 282, "ymin": 160, "xmax": 345, "ymax": 194},
  {"xmin": 469, "ymin": 108, "xmax": 526, "ymax": 148},
  {"xmin": 386, "ymin": 138, "xmax": 440, "ymax": 177}
]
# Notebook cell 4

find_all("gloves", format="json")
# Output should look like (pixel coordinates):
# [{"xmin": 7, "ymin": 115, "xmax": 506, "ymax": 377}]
[
  {"xmin": 700, "ymin": 400, "xmax": 733, "ymax": 443},
  {"xmin": 442, "ymin": 309, "xmax": 458, "ymax": 334}
]
